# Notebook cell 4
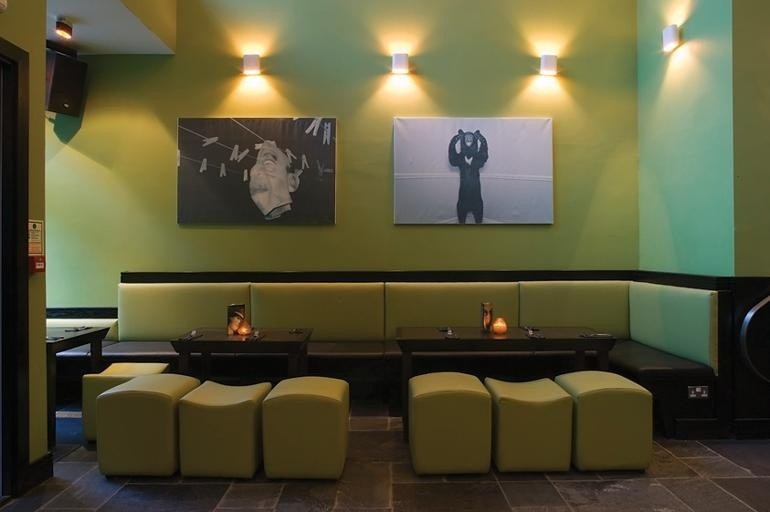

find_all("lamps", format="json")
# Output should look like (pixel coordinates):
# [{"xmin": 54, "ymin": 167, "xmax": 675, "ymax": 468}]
[
  {"xmin": 662, "ymin": 25, "xmax": 681, "ymax": 54},
  {"xmin": 55, "ymin": 17, "xmax": 73, "ymax": 41},
  {"xmin": 242, "ymin": 54, "xmax": 261, "ymax": 75},
  {"xmin": 540, "ymin": 55, "xmax": 558, "ymax": 75},
  {"xmin": 392, "ymin": 53, "xmax": 409, "ymax": 74}
]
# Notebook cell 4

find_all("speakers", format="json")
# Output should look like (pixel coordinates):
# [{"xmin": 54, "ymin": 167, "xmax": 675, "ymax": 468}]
[{"xmin": 46, "ymin": 44, "xmax": 88, "ymax": 118}]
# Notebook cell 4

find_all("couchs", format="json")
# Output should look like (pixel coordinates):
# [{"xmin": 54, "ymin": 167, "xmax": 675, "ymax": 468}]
[{"xmin": 45, "ymin": 271, "xmax": 732, "ymax": 438}]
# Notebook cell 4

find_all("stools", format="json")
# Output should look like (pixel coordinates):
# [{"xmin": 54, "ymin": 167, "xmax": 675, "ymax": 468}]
[
  {"xmin": 81, "ymin": 363, "xmax": 170, "ymax": 451},
  {"xmin": 262, "ymin": 376, "xmax": 350, "ymax": 479},
  {"xmin": 554, "ymin": 369, "xmax": 655, "ymax": 472},
  {"xmin": 95, "ymin": 374, "xmax": 201, "ymax": 477},
  {"xmin": 483, "ymin": 376, "xmax": 573, "ymax": 474},
  {"xmin": 177, "ymin": 380, "xmax": 272, "ymax": 478},
  {"xmin": 407, "ymin": 371, "xmax": 492, "ymax": 477}
]
